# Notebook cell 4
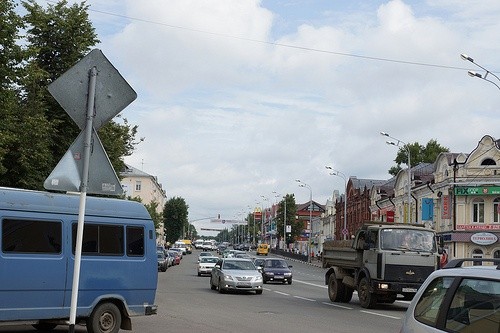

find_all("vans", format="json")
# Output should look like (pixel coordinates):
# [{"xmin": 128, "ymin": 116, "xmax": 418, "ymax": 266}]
[
  {"xmin": 203, "ymin": 241, "xmax": 212, "ymax": 251},
  {"xmin": 0, "ymin": 185, "xmax": 159, "ymax": 333},
  {"xmin": 182, "ymin": 240, "xmax": 192, "ymax": 254},
  {"xmin": 175, "ymin": 242, "xmax": 187, "ymax": 255},
  {"xmin": 195, "ymin": 239, "xmax": 204, "ymax": 249}
]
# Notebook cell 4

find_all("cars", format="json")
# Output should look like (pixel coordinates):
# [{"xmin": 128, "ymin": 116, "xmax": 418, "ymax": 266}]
[
  {"xmin": 198, "ymin": 252, "xmax": 220, "ymax": 276},
  {"xmin": 253, "ymin": 258, "xmax": 293, "ymax": 284},
  {"xmin": 155, "ymin": 246, "xmax": 183, "ymax": 272},
  {"xmin": 210, "ymin": 258, "xmax": 264, "ymax": 294},
  {"xmin": 214, "ymin": 241, "xmax": 268, "ymax": 262}
]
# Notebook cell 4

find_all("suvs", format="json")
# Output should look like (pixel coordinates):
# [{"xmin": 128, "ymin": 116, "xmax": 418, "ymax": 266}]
[{"xmin": 401, "ymin": 258, "xmax": 500, "ymax": 333}]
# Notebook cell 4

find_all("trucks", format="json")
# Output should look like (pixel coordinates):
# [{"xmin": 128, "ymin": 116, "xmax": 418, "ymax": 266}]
[{"xmin": 321, "ymin": 221, "xmax": 446, "ymax": 307}]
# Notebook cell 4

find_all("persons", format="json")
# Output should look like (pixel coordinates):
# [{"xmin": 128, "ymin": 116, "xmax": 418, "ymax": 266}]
[
  {"xmin": 441, "ymin": 250, "xmax": 447, "ymax": 267},
  {"xmin": 292, "ymin": 247, "xmax": 314, "ymax": 261},
  {"xmin": 317, "ymin": 251, "xmax": 322, "ymax": 263}
]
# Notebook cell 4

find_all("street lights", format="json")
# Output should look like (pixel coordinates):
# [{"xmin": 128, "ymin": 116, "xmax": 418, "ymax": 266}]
[
  {"xmin": 380, "ymin": 132, "xmax": 411, "ymax": 222},
  {"xmin": 272, "ymin": 191, "xmax": 286, "ymax": 252},
  {"xmin": 238, "ymin": 195, "xmax": 270, "ymax": 244},
  {"xmin": 295, "ymin": 179, "xmax": 313, "ymax": 263},
  {"xmin": 325, "ymin": 165, "xmax": 347, "ymax": 240}
]
